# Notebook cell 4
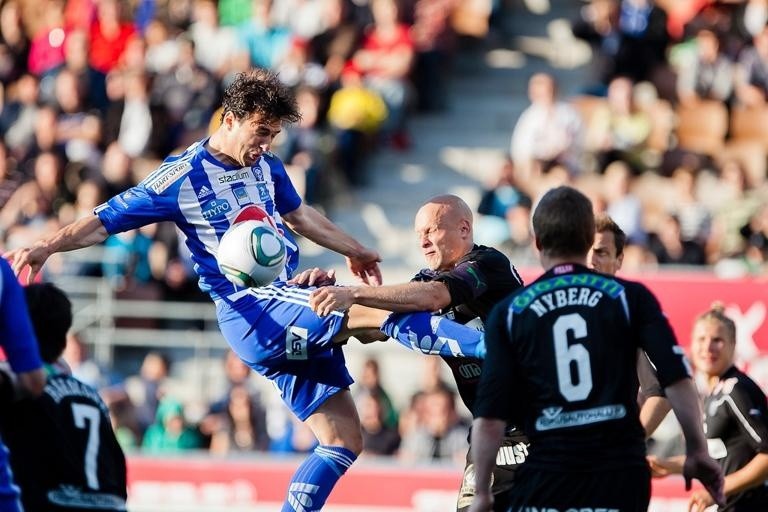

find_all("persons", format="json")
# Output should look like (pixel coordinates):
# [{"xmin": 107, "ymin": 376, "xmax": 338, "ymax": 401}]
[{"xmin": 0, "ymin": 1, "xmax": 767, "ymax": 511}]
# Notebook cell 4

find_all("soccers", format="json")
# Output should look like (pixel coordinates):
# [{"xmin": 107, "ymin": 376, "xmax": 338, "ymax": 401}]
[{"xmin": 217, "ymin": 219, "xmax": 287, "ymax": 288}]
